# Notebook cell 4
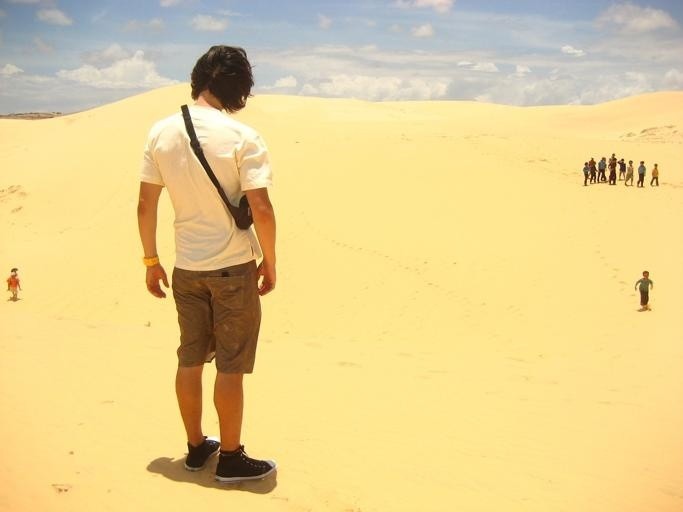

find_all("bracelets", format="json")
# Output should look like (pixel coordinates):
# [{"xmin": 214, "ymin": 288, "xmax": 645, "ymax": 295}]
[{"xmin": 143, "ymin": 256, "xmax": 159, "ymax": 267}]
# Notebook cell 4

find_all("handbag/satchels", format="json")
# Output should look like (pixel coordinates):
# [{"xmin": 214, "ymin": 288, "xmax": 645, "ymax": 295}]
[{"xmin": 237, "ymin": 195, "xmax": 253, "ymax": 231}]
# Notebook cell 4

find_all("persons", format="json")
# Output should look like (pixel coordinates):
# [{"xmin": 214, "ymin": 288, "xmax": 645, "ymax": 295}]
[
  {"xmin": 625, "ymin": 160, "xmax": 634, "ymax": 186},
  {"xmin": 637, "ymin": 160, "xmax": 646, "ymax": 187},
  {"xmin": 137, "ymin": 43, "xmax": 278, "ymax": 479},
  {"xmin": 7, "ymin": 268, "xmax": 23, "ymax": 301},
  {"xmin": 582, "ymin": 153, "xmax": 626, "ymax": 185},
  {"xmin": 649, "ymin": 164, "xmax": 659, "ymax": 186},
  {"xmin": 634, "ymin": 270, "xmax": 654, "ymax": 312}
]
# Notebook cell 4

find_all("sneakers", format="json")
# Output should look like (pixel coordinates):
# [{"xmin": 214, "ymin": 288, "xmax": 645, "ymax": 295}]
[
  {"xmin": 184, "ymin": 437, "xmax": 221, "ymax": 472},
  {"xmin": 215, "ymin": 445, "xmax": 276, "ymax": 483}
]
